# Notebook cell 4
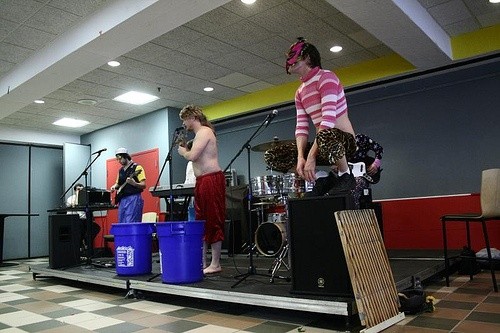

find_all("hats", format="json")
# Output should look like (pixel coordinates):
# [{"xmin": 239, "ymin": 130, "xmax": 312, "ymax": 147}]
[{"xmin": 116, "ymin": 148, "xmax": 128, "ymax": 154}]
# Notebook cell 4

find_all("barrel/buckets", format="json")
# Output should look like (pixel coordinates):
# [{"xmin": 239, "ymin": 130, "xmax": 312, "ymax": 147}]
[
  {"xmin": 110, "ymin": 222, "xmax": 158, "ymax": 277},
  {"xmin": 156, "ymin": 221, "xmax": 207, "ymax": 286}
]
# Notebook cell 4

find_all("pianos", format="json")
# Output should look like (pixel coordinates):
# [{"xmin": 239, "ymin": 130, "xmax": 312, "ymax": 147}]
[{"xmin": 148, "ymin": 182, "xmax": 196, "ymax": 221}]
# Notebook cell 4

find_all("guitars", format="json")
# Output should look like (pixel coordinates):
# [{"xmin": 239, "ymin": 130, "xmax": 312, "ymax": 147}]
[
  {"xmin": 337, "ymin": 155, "xmax": 381, "ymax": 184},
  {"xmin": 114, "ymin": 169, "xmax": 142, "ymax": 205}
]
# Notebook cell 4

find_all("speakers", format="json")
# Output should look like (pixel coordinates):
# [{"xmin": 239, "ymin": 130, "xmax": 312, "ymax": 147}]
[
  {"xmin": 291, "ymin": 196, "xmax": 355, "ymax": 298},
  {"xmin": 49, "ymin": 214, "xmax": 80, "ymax": 269}
]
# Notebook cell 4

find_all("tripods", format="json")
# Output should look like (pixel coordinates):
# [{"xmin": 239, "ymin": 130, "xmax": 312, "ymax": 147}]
[
  {"xmin": 223, "ymin": 114, "xmax": 291, "ymax": 288},
  {"xmin": 59, "ymin": 157, "xmax": 109, "ymax": 270}
]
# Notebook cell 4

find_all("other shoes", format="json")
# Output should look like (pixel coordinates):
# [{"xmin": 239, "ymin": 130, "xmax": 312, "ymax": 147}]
[
  {"xmin": 83, "ymin": 239, "xmax": 88, "ymax": 249},
  {"xmin": 203, "ymin": 262, "xmax": 223, "ymax": 274},
  {"xmin": 304, "ymin": 171, "xmax": 336, "ymax": 196},
  {"xmin": 327, "ymin": 171, "xmax": 357, "ymax": 195},
  {"xmin": 79, "ymin": 246, "xmax": 83, "ymax": 252}
]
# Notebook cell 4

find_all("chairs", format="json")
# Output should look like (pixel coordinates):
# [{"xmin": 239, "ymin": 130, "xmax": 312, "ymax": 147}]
[{"xmin": 439, "ymin": 167, "xmax": 500, "ymax": 295}]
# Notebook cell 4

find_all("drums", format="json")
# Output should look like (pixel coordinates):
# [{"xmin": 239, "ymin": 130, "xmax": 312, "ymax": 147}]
[
  {"xmin": 284, "ymin": 174, "xmax": 306, "ymax": 195},
  {"xmin": 271, "ymin": 195, "xmax": 286, "ymax": 208},
  {"xmin": 255, "ymin": 220, "xmax": 288, "ymax": 257},
  {"xmin": 267, "ymin": 213, "xmax": 286, "ymax": 223},
  {"xmin": 305, "ymin": 170, "xmax": 319, "ymax": 192},
  {"xmin": 252, "ymin": 176, "xmax": 284, "ymax": 195}
]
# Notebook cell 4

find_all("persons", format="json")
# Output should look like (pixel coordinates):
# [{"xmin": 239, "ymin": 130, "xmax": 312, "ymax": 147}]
[
  {"xmin": 339, "ymin": 136, "xmax": 385, "ymax": 198},
  {"xmin": 65, "ymin": 183, "xmax": 101, "ymax": 254},
  {"xmin": 110, "ymin": 146, "xmax": 147, "ymax": 224},
  {"xmin": 264, "ymin": 35, "xmax": 355, "ymax": 197},
  {"xmin": 175, "ymin": 104, "xmax": 226, "ymax": 273}
]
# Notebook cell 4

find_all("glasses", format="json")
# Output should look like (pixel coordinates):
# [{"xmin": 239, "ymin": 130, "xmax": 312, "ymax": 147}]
[
  {"xmin": 77, "ymin": 188, "xmax": 80, "ymax": 190},
  {"xmin": 119, "ymin": 157, "xmax": 122, "ymax": 160}
]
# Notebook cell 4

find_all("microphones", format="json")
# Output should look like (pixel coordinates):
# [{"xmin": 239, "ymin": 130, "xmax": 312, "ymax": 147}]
[
  {"xmin": 175, "ymin": 126, "xmax": 186, "ymax": 133},
  {"xmin": 92, "ymin": 148, "xmax": 108, "ymax": 155},
  {"xmin": 275, "ymin": 196, "xmax": 284, "ymax": 203},
  {"xmin": 265, "ymin": 110, "xmax": 278, "ymax": 127}
]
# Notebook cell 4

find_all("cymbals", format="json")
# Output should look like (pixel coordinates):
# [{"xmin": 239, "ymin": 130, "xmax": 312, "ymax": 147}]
[
  {"xmin": 251, "ymin": 140, "xmax": 294, "ymax": 153},
  {"xmin": 252, "ymin": 202, "xmax": 273, "ymax": 205}
]
